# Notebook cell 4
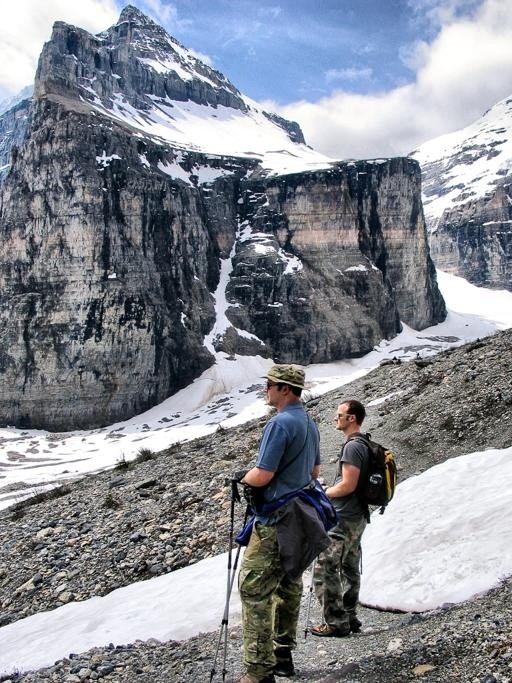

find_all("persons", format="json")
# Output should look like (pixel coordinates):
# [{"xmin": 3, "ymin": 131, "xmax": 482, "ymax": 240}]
[
  {"xmin": 311, "ymin": 395, "xmax": 376, "ymax": 639},
  {"xmin": 232, "ymin": 360, "xmax": 322, "ymax": 682}
]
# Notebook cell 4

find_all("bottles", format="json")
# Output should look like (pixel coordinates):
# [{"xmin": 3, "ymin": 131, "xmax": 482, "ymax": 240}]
[{"xmin": 371, "ymin": 475, "xmax": 382, "ymax": 484}]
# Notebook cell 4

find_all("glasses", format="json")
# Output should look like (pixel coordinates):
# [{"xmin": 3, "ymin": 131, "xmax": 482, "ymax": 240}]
[
  {"xmin": 336, "ymin": 413, "xmax": 352, "ymax": 416},
  {"xmin": 262, "ymin": 380, "xmax": 277, "ymax": 389}
]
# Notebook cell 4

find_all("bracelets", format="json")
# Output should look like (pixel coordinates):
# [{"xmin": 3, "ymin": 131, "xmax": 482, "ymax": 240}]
[{"xmin": 324, "ymin": 485, "xmax": 330, "ymax": 491}]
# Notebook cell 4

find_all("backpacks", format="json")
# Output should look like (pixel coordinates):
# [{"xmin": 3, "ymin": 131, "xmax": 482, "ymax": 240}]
[{"xmin": 363, "ymin": 438, "xmax": 397, "ymax": 508}]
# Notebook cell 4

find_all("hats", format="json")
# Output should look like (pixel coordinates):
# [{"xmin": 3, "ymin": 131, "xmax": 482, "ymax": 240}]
[{"xmin": 261, "ymin": 365, "xmax": 313, "ymax": 392}]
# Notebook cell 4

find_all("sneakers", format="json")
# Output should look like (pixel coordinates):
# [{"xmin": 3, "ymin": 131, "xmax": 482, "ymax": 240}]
[
  {"xmin": 227, "ymin": 670, "xmax": 277, "ymax": 682},
  {"xmin": 274, "ymin": 663, "xmax": 296, "ymax": 676},
  {"xmin": 311, "ymin": 622, "xmax": 348, "ymax": 636}
]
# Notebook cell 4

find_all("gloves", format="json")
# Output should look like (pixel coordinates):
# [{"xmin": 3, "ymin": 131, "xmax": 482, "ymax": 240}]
[{"xmin": 233, "ymin": 469, "xmax": 251, "ymax": 485}]
